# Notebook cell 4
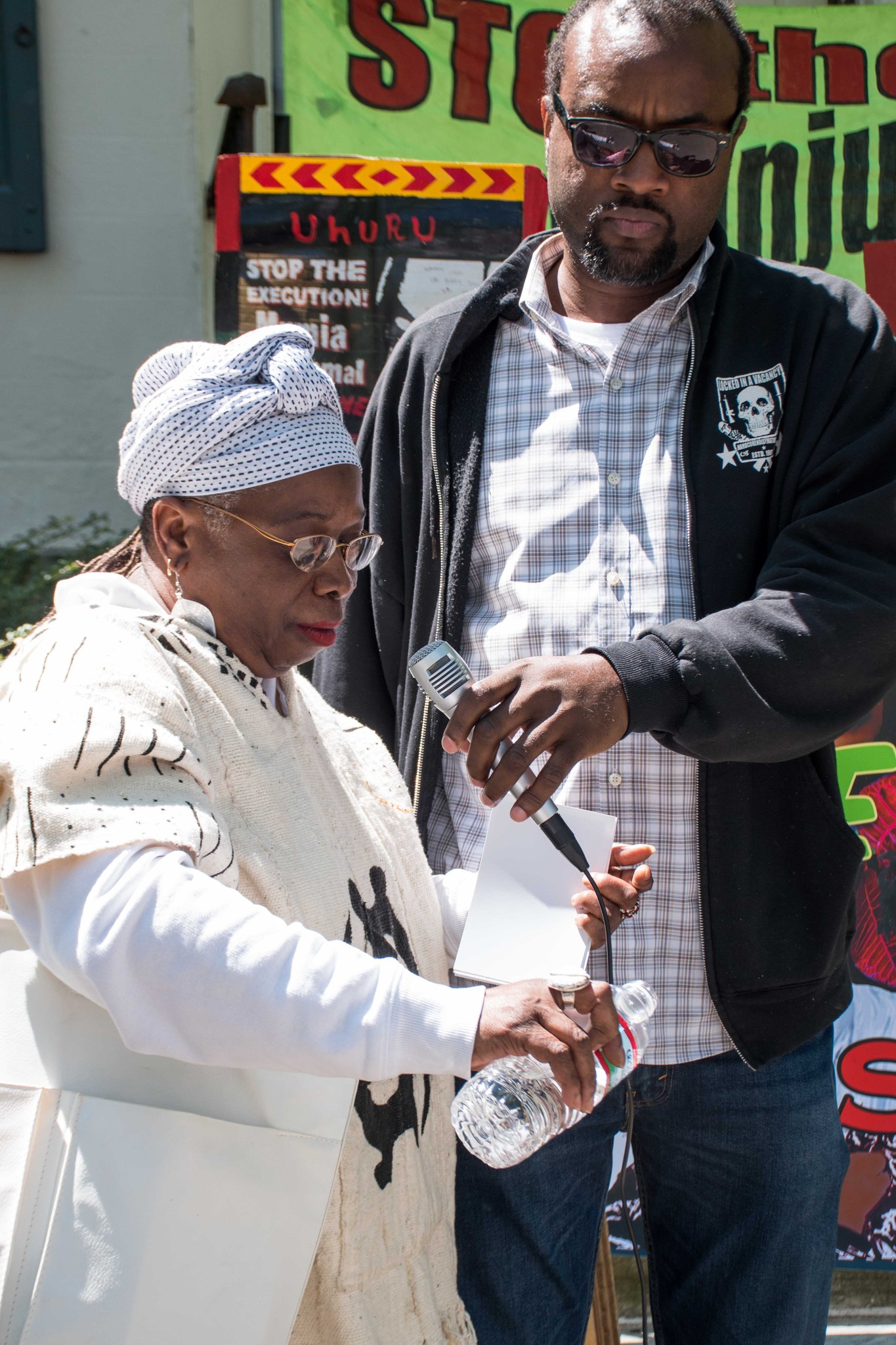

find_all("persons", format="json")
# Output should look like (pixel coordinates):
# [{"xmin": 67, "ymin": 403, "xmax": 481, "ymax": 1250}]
[
  {"xmin": 0, "ymin": 322, "xmax": 653, "ymax": 1345},
  {"xmin": 311, "ymin": 1, "xmax": 896, "ymax": 1345}
]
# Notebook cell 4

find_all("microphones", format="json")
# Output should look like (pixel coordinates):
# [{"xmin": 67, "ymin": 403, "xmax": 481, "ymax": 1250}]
[{"xmin": 406, "ymin": 639, "xmax": 590, "ymax": 873}]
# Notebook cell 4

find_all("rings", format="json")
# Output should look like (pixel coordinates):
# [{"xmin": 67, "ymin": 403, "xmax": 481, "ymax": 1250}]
[
  {"xmin": 621, "ymin": 898, "xmax": 640, "ymax": 919},
  {"xmin": 547, "ymin": 966, "xmax": 590, "ymax": 1009}
]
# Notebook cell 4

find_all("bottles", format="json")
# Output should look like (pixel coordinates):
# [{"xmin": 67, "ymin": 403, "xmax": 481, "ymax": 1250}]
[{"xmin": 450, "ymin": 980, "xmax": 658, "ymax": 1169}]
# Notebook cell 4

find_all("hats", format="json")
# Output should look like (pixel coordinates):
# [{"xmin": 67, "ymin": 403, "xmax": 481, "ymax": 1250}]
[{"xmin": 116, "ymin": 323, "xmax": 358, "ymax": 517}]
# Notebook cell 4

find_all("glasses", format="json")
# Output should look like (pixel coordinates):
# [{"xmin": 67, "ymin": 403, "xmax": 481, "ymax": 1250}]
[
  {"xmin": 181, "ymin": 494, "xmax": 383, "ymax": 576},
  {"xmin": 550, "ymin": 92, "xmax": 746, "ymax": 179}
]
